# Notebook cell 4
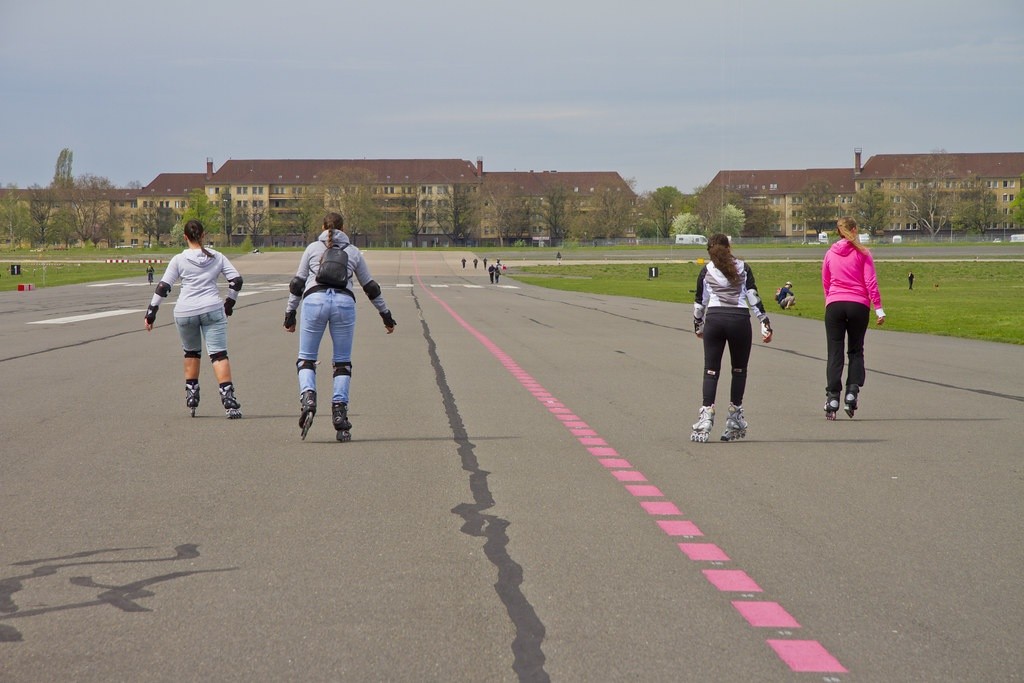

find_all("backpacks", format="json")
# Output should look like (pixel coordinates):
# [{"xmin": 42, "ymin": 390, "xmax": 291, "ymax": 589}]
[{"xmin": 309, "ymin": 241, "xmax": 352, "ymax": 287}]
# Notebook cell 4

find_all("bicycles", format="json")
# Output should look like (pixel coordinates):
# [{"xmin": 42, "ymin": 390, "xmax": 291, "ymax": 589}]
[{"xmin": 148, "ymin": 274, "xmax": 153, "ymax": 284}]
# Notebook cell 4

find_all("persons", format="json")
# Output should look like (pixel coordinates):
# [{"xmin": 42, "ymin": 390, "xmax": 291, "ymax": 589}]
[
  {"xmin": 144, "ymin": 220, "xmax": 243, "ymax": 409},
  {"xmin": 282, "ymin": 212, "xmax": 397, "ymax": 432},
  {"xmin": 691, "ymin": 233, "xmax": 773, "ymax": 431},
  {"xmin": 907, "ymin": 271, "xmax": 915, "ymax": 289},
  {"xmin": 778, "ymin": 281, "xmax": 796, "ymax": 310},
  {"xmin": 461, "ymin": 256, "xmax": 502, "ymax": 284},
  {"xmin": 822, "ymin": 217, "xmax": 886, "ymax": 411},
  {"xmin": 146, "ymin": 265, "xmax": 154, "ymax": 281}
]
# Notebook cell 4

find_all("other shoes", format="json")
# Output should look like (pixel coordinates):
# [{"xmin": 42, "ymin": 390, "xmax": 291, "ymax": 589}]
[
  {"xmin": 782, "ymin": 306, "xmax": 785, "ymax": 310},
  {"xmin": 785, "ymin": 308, "xmax": 791, "ymax": 310},
  {"xmin": 775, "ymin": 288, "xmax": 782, "ymax": 301}
]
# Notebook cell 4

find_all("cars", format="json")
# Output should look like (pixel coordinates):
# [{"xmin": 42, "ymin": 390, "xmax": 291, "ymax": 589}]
[{"xmin": 993, "ymin": 238, "xmax": 1001, "ymax": 242}]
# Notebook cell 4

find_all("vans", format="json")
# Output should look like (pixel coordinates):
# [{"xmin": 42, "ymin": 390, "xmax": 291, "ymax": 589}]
[{"xmin": 675, "ymin": 234, "xmax": 708, "ymax": 245}]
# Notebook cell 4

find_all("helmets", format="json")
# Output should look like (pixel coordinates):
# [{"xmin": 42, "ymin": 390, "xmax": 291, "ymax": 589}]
[{"xmin": 786, "ymin": 281, "xmax": 792, "ymax": 287}]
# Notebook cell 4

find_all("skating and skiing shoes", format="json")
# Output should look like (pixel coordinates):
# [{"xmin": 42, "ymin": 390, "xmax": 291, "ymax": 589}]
[
  {"xmin": 219, "ymin": 385, "xmax": 242, "ymax": 419},
  {"xmin": 720, "ymin": 402, "xmax": 748, "ymax": 441},
  {"xmin": 844, "ymin": 384, "xmax": 859, "ymax": 418},
  {"xmin": 332, "ymin": 402, "xmax": 352, "ymax": 442},
  {"xmin": 299, "ymin": 391, "xmax": 316, "ymax": 440},
  {"xmin": 185, "ymin": 384, "xmax": 200, "ymax": 417},
  {"xmin": 690, "ymin": 404, "xmax": 715, "ymax": 443},
  {"xmin": 824, "ymin": 392, "xmax": 840, "ymax": 421}
]
